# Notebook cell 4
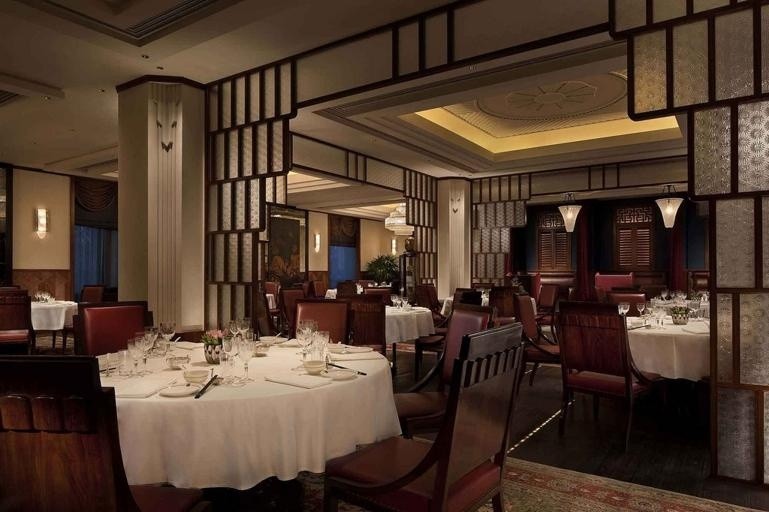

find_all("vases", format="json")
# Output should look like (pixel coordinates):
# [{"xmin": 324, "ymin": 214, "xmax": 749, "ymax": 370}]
[{"xmin": 204, "ymin": 344, "xmax": 222, "ymax": 364}]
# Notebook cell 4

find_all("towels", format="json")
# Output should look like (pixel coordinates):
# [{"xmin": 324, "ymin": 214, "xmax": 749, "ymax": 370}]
[
  {"xmin": 264, "ymin": 375, "xmax": 331, "ymax": 390},
  {"xmin": 329, "ymin": 352, "xmax": 384, "ymax": 361},
  {"xmin": 175, "ymin": 341, "xmax": 203, "ymax": 349},
  {"xmin": 115, "ymin": 376, "xmax": 176, "ymax": 398},
  {"xmin": 280, "ymin": 339, "xmax": 302, "ymax": 346}
]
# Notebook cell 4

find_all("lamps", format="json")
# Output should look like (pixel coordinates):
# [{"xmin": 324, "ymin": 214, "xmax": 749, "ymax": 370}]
[
  {"xmin": 654, "ymin": 183, "xmax": 685, "ymax": 229},
  {"xmin": 385, "ymin": 203, "xmax": 415, "ymax": 236},
  {"xmin": 33, "ymin": 207, "xmax": 51, "ymax": 238},
  {"xmin": 558, "ymin": 193, "xmax": 583, "ymax": 233}
]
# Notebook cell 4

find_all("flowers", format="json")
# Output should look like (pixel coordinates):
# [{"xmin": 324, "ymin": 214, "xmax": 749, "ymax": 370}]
[{"xmin": 200, "ymin": 329, "xmax": 228, "ymax": 345}]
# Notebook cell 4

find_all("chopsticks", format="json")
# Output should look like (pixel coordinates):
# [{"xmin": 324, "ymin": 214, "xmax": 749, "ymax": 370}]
[
  {"xmin": 195, "ymin": 375, "xmax": 218, "ymax": 399},
  {"xmin": 328, "ymin": 362, "xmax": 366, "ymax": 376}
]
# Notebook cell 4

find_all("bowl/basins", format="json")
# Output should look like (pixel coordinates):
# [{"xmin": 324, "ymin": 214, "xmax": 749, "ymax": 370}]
[
  {"xmin": 253, "ymin": 343, "xmax": 271, "ymax": 358},
  {"xmin": 327, "ymin": 343, "xmax": 345, "ymax": 353},
  {"xmin": 183, "ymin": 368, "xmax": 209, "ymax": 383}
]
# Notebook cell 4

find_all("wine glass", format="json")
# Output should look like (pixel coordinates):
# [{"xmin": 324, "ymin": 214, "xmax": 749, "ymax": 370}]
[
  {"xmin": 297, "ymin": 320, "xmax": 332, "ymax": 375},
  {"xmin": 104, "ymin": 320, "xmax": 176, "ymax": 379},
  {"xmin": 617, "ymin": 302, "xmax": 630, "ymax": 331},
  {"xmin": 636, "ymin": 289, "xmax": 711, "ymax": 329},
  {"xmin": 34, "ymin": 291, "xmax": 55, "ymax": 303},
  {"xmin": 220, "ymin": 318, "xmax": 256, "ymax": 388},
  {"xmin": 390, "ymin": 294, "xmax": 409, "ymax": 311}
]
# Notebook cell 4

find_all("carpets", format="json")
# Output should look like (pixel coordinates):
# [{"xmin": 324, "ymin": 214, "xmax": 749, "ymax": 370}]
[{"xmin": 301, "ymin": 432, "xmax": 765, "ymax": 512}]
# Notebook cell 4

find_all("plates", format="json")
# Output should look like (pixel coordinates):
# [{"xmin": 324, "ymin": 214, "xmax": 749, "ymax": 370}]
[
  {"xmin": 161, "ymin": 385, "xmax": 200, "ymax": 397},
  {"xmin": 348, "ymin": 346, "xmax": 374, "ymax": 352},
  {"xmin": 260, "ymin": 336, "xmax": 288, "ymax": 344},
  {"xmin": 330, "ymin": 369, "xmax": 356, "ymax": 379},
  {"xmin": 279, "ymin": 338, "xmax": 299, "ymax": 347}
]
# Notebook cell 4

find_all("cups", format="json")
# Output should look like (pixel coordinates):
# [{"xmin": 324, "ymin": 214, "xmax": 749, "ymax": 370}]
[
  {"xmin": 367, "ymin": 281, "xmax": 393, "ymax": 287},
  {"xmin": 167, "ymin": 353, "xmax": 190, "ymax": 370}
]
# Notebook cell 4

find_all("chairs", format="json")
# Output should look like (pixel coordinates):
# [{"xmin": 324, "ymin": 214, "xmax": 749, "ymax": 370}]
[
  {"xmin": 252, "ymin": 271, "xmax": 667, "ymax": 452},
  {"xmin": 1, "ymin": 355, "xmax": 212, "ymax": 511},
  {"xmin": 63, "ymin": 285, "xmax": 107, "ymax": 353},
  {"xmin": 78, "ymin": 301, "xmax": 150, "ymax": 357},
  {"xmin": 686, "ymin": 270, "xmax": 710, "ymax": 299},
  {"xmin": 0, "ymin": 286, "xmax": 21, "ymax": 289},
  {"xmin": 0, "ymin": 290, "xmax": 33, "ymax": 355},
  {"xmin": 324, "ymin": 323, "xmax": 524, "ymax": 511}
]
